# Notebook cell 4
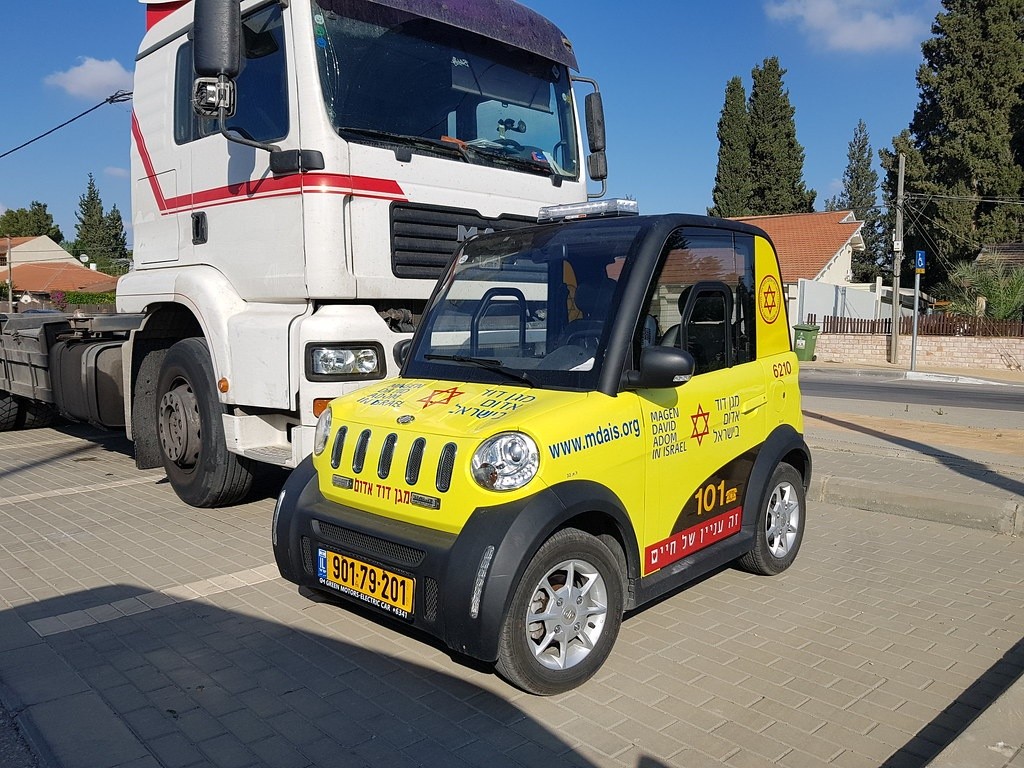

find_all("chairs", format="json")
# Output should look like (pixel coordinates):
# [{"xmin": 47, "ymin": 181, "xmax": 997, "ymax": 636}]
[
  {"xmin": 660, "ymin": 280, "xmax": 746, "ymax": 375},
  {"xmin": 558, "ymin": 278, "xmax": 617, "ymax": 347}
]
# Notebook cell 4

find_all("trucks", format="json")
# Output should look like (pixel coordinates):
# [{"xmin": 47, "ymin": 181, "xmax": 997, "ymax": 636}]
[{"xmin": 0, "ymin": 0, "xmax": 608, "ymax": 508}]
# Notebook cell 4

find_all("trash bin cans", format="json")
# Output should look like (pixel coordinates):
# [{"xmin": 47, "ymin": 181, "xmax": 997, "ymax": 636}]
[{"xmin": 792, "ymin": 323, "xmax": 820, "ymax": 361}]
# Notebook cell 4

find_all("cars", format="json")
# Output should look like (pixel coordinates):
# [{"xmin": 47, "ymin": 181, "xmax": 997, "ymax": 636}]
[{"xmin": 272, "ymin": 198, "xmax": 812, "ymax": 696}]
